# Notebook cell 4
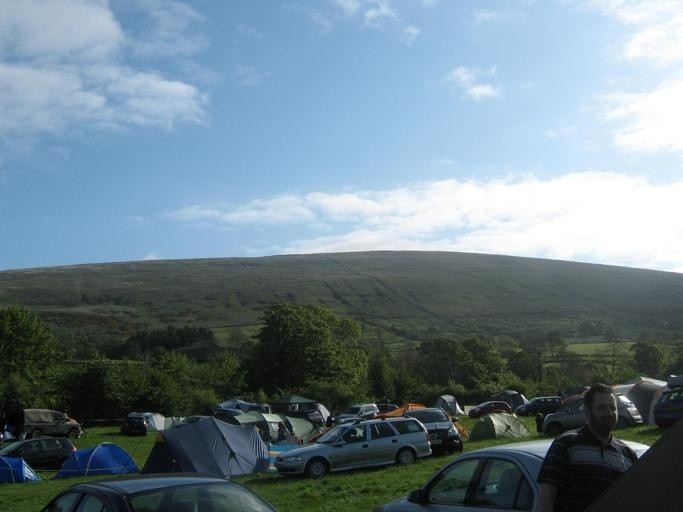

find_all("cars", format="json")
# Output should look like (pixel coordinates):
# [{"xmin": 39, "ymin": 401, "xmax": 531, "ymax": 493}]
[
  {"xmin": 184, "ymin": 415, "xmax": 210, "ymax": 424},
  {"xmin": 370, "ymin": 437, "xmax": 653, "ymax": 511},
  {"xmin": 120, "ymin": 415, "xmax": 148, "ymax": 437},
  {"xmin": 38, "ymin": 476, "xmax": 281, "ymax": 511},
  {"xmin": 468, "ymin": 400, "xmax": 512, "ymax": 418}
]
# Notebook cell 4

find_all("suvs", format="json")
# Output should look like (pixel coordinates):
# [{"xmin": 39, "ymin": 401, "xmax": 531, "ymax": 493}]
[
  {"xmin": 274, "ymin": 403, "xmax": 464, "ymax": 476},
  {"xmin": 212, "ymin": 408, "xmax": 245, "ymax": 427},
  {"xmin": 652, "ymin": 374, "xmax": 682, "ymax": 428},
  {"xmin": 538, "ymin": 392, "xmax": 642, "ymax": 434}
]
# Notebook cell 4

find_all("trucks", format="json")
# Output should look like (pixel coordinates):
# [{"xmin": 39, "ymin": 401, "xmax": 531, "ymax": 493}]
[{"xmin": 12, "ymin": 409, "xmax": 81, "ymax": 438}]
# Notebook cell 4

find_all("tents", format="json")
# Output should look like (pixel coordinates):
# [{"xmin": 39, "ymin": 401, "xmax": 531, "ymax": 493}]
[
  {"xmin": 52, "ymin": 441, "xmax": 140, "ymax": 480},
  {"xmin": 611, "ymin": 375, "xmax": 669, "ymax": 425},
  {"xmin": 216, "ymin": 394, "xmax": 331, "ymax": 444},
  {"xmin": 0, "ymin": 455, "xmax": 42, "ymax": 484},
  {"xmin": 469, "ymin": 412, "xmax": 532, "ymax": 439},
  {"xmin": 144, "ymin": 412, "xmax": 165, "ymax": 431},
  {"xmin": 435, "ymin": 394, "xmax": 464, "ymax": 415},
  {"xmin": 377, "ymin": 403, "xmax": 468, "ymax": 439},
  {"xmin": 489, "ymin": 389, "xmax": 528, "ymax": 411},
  {"xmin": 141, "ymin": 415, "xmax": 270, "ymax": 477}
]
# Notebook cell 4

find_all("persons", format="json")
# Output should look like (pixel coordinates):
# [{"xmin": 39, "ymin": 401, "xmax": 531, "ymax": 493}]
[{"xmin": 533, "ymin": 382, "xmax": 637, "ymax": 512}]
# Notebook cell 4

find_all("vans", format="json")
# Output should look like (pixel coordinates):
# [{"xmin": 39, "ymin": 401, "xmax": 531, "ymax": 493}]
[
  {"xmin": 515, "ymin": 395, "xmax": 563, "ymax": 418},
  {"xmin": 0, "ymin": 438, "xmax": 78, "ymax": 469}
]
